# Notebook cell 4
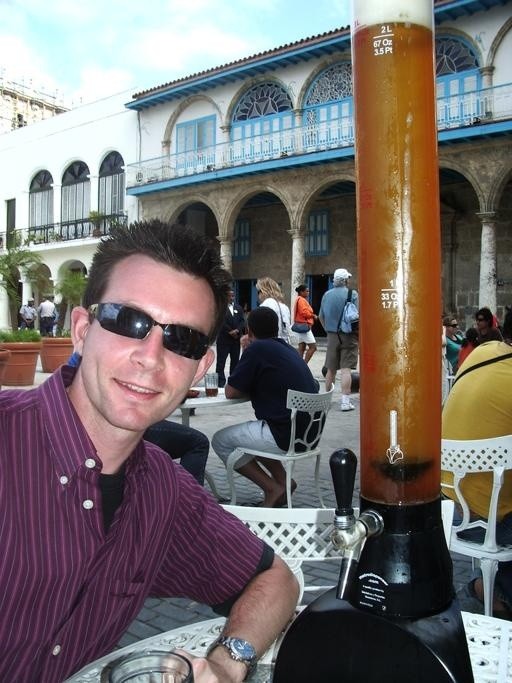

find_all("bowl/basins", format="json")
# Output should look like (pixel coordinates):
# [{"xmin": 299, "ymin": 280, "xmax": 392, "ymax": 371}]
[{"xmin": 186, "ymin": 390, "xmax": 200, "ymax": 399}]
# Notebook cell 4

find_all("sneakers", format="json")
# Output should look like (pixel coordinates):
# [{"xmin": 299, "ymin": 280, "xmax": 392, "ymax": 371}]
[{"xmin": 341, "ymin": 402, "xmax": 355, "ymax": 411}]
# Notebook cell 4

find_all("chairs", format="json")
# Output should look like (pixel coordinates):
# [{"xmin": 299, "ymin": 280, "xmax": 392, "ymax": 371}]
[
  {"xmin": 225, "ymin": 382, "xmax": 335, "ymax": 508},
  {"xmin": 406, "ymin": 435, "xmax": 512, "ymax": 627},
  {"xmin": 217, "ymin": 504, "xmax": 380, "ymax": 623}
]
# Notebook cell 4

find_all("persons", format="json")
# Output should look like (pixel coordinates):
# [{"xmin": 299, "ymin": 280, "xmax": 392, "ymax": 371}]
[
  {"xmin": 211, "ymin": 306, "xmax": 326, "ymax": 508},
  {"xmin": 440, "ymin": 309, "xmax": 512, "ymax": 621},
  {"xmin": 36, "ymin": 294, "xmax": 56, "ymax": 338},
  {"xmin": 19, "ymin": 298, "xmax": 37, "ymax": 330},
  {"xmin": 318, "ymin": 269, "xmax": 359, "ymax": 411},
  {"xmin": 67, "ymin": 351, "xmax": 209, "ymax": 487},
  {"xmin": 215, "ymin": 277, "xmax": 319, "ymax": 388},
  {"xmin": 442, "ymin": 306, "xmax": 503, "ymax": 376},
  {"xmin": 0, "ymin": 218, "xmax": 299, "ymax": 683}
]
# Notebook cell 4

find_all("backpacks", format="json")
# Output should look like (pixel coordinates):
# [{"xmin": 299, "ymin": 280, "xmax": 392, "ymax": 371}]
[{"xmin": 336, "ymin": 289, "xmax": 360, "ymax": 336}]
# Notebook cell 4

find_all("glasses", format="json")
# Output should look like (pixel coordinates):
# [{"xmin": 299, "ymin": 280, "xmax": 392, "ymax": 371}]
[
  {"xmin": 445, "ymin": 323, "xmax": 459, "ymax": 328},
  {"xmin": 477, "ymin": 318, "xmax": 484, "ymax": 322},
  {"xmin": 88, "ymin": 302, "xmax": 212, "ymax": 361}
]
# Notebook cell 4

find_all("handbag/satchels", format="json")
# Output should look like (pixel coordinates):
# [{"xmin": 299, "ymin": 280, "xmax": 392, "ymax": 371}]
[{"xmin": 292, "ymin": 322, "xmax": 311, "ymax": 333}]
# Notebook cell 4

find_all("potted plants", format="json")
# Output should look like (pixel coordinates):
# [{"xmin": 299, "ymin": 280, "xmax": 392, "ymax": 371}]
[{"xmin": 0, "ymin": 212, "xmax": 125, "ymax": 387}]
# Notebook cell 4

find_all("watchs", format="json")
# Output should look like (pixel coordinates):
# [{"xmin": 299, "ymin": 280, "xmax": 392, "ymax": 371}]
[{"xmin": 206, "ymin": 635, "xmax": 257, "ymax": 682}]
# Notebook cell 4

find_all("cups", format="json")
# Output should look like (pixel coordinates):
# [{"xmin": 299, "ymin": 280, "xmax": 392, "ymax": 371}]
[
  {"xmin": 203, "ymin": 372, "xmax": 219, "ymax": 397},
  {"xmin": 108, "ymin": 648, "xmax": 196, "ymax": 683}
]
